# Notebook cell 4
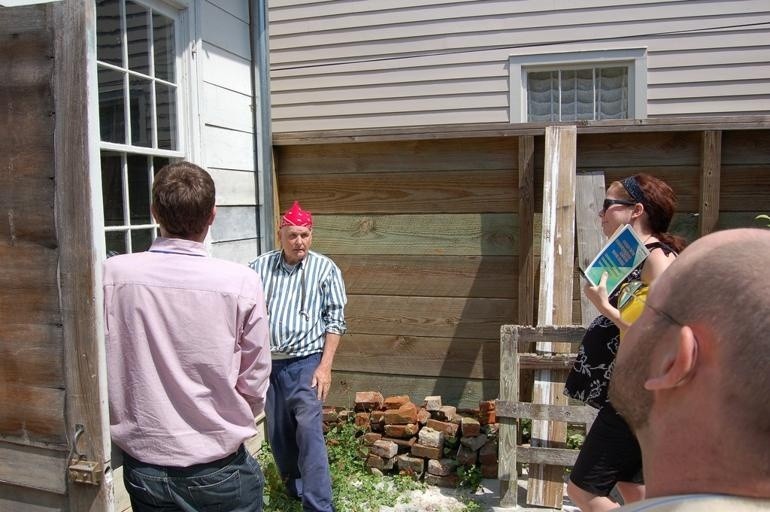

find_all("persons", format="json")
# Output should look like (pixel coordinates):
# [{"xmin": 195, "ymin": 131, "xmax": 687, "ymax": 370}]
[
  {"xmin": 610, "ymin": 226, "xmax": 770, "ymax": 512},
  {"xmin": 97, "ymin": 161, "xmax": 274, "ymax": 512},
  {"xmin": 567, "ymin": 172, "xmax": 681, "ymax": 512},
  {"xmin": 247, "ymin": 203, "xmax": 348, "ymax": 512}
]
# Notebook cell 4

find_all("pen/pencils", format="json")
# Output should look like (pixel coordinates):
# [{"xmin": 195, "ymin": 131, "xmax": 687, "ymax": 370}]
[{"xmin": 577, "ymin": 267, "xmax": 585, "ymax": 275}]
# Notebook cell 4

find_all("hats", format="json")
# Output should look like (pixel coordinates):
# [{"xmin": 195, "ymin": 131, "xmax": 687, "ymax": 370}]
[{"xmin": 279, "ymin": 201, "xmax": 313, "ymax": 230}]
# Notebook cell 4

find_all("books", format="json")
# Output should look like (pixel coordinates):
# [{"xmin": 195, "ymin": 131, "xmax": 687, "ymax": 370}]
[{"xmin": 583, "ymin": 224, "xmax": 649, "ymax": 298}]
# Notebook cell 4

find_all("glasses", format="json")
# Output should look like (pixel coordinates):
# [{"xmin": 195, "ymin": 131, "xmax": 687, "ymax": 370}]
[
  {"xmin": 601, "ymin": 197, "xmax": 637, "ymax": 213},
  {"xmin": 615, "ymin": 279, "xmax": 685, "ymax": 335}
]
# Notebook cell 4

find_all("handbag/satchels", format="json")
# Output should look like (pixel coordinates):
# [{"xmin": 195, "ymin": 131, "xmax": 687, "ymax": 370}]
[{"xmin": 562, "ymin": 315, "xmax": 618, "ymax": 409}]
